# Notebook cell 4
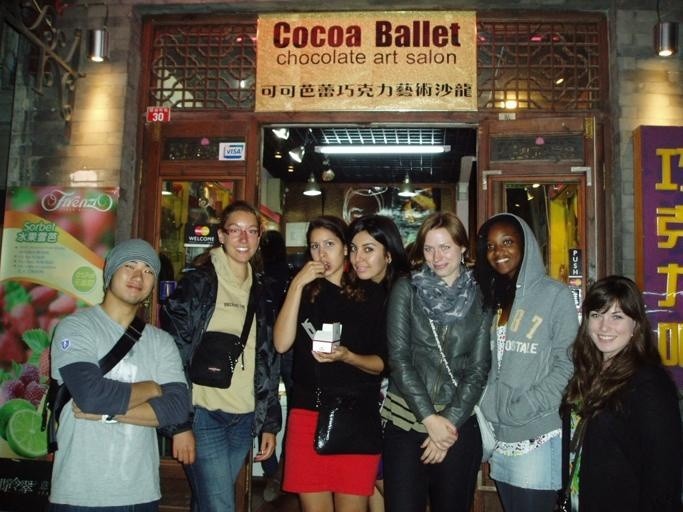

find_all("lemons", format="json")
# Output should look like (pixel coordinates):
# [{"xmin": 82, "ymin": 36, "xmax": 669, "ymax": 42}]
[{"xmin": 1, "ymin": 399, "xmax": 36, "ymax": 439}]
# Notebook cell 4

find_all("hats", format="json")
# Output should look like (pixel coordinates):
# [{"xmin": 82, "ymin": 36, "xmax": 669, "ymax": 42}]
[{"xmin": 103, "ymin": 238, "xmax": 163, "ymax": 291}]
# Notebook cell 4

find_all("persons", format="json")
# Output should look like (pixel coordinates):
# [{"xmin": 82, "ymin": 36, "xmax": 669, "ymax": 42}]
[
  {"xmin": 273, "ymin": 214, "xmax": 388, "ymax": 511},
  {"xmin": 559, "ymin": 274, "xmax": 682, "ymax": 512},
  {"xmin": 159, "ymin": 199, "xmax": 282, "ymax": 512},
  {"xmin": 474, "ymin": 212, "xmax": 579, "ymax": 512},
  {"xmin": 47, "ymin": 238, "xmax": 190, "ymax": 511},
  {"xmin": 343, "ymin": 214, "xmax": 410, "ymax": 512},
  {"xmin": 379, "ymin": 210, "xmax": 493, "ymax": 512}
]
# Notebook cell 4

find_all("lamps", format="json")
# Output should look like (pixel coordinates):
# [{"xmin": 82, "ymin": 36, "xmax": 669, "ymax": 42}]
[
  {"xmin": 301, "ymin": 170, "xmax": 321, "ymax": 197},
  {"xmin": 522, "ymin": 184, "xmax": 536, "ymax": 202},
  {"xmin": 648, "ymin": 1, "xmax": 679, "ymax": 61},
  {"xmin": 161, "ymin": 178, "xmax": 174, "ymax": 196},
  {"xmin": 85, "ymin": 2, "xmax": 113, "ymax": 65},
  {"xmin": 318, "ymin": 157, "xmax": 336, "ymax": 183},
  {"xmin": 271, "ymin": 127, "xmax": 314, "ymax": 164},
  {"xmin": 311, "ymin": 129, "xmax": 452, "ymax": 158},
  {"xmin": 395, "ymin": 171, "xmax": 419, "ymax": 198}
]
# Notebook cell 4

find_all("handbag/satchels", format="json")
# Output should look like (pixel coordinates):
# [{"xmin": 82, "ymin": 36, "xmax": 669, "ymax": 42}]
[
  {"xmin": 472, "ymin": 403, "xmax": 497, "ymax": 466},
  {"xmin": 313, "ymin": 387, "xmax": 382, "ymax": 455},
  {"xmin": 188, "ymin": 330, "xmax": 245, "ymax": 389}
]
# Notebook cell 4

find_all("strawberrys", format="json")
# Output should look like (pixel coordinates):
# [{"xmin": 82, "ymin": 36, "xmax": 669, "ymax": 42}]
[{"xmin": 0, "ymin": 280, "xmax": 77, "ymax": 404}]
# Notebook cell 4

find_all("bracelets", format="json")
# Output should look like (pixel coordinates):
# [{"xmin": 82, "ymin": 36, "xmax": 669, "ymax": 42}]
[{"xmin": 106, "ymin": 415, "xmax": 114, "ymax": 421}]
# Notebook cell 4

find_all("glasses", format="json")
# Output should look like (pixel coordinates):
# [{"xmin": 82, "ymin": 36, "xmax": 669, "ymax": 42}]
[{"xmin": 222, "ymin": 226, "xmax": 260, "ymax": 238}]
